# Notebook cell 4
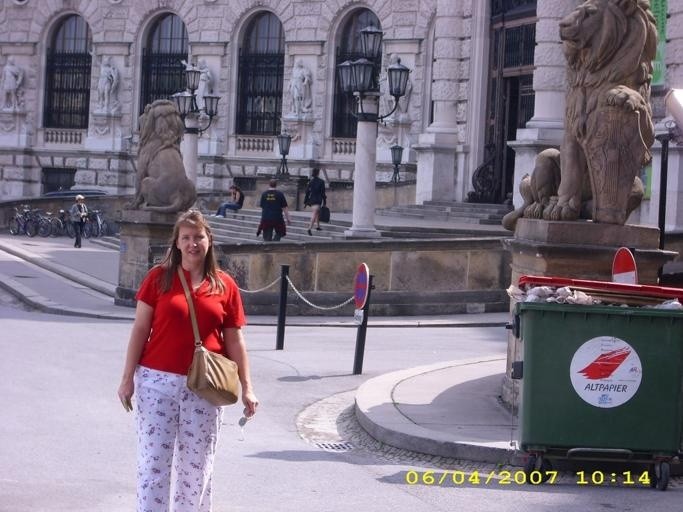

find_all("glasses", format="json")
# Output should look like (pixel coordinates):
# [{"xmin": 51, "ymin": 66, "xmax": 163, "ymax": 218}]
[{"xmin": 238, "ymin": 408, "xmax": 255, "ymax": 441}]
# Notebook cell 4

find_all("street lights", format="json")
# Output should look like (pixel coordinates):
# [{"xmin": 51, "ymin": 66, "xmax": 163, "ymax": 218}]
[
  {"xmin": 173, "ymin": 58, "xmax": 219, "ymax": 190},
  {"xmin": 386, "ymin": 142, "xmax": 405, "ymax": 183},
  {"xmin": 650, "ymin": 118, "xmax": 672, "ymax": 286},
  {"xmin": 276, "ymin": 129, "xmax": 291, "ymax": 180},
  {"xmin": 336, "ymin": 20, "xmax": 409, "ymax": 241}
]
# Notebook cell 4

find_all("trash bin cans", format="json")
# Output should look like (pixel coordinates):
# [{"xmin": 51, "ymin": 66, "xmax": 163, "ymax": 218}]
[{"xmin": 509, "ymin": 275, "xmax": 683, "ymax": 491}]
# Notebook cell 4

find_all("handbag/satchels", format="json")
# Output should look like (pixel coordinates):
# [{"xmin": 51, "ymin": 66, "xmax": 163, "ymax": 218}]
[
  {"xmin": 187, "ymin": 347, "xmax": 239, "ymax": 405},
  {"xmin": 319, "ymin": 206, "xmax": 330, "ymax": 222}
]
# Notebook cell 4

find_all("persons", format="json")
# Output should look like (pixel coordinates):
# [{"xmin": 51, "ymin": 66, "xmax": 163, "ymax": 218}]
[
  {"xmin": 209, "ymin": 185, "xmax": 245, "ymax": 219},
  {"xmin": 117, "ymin": 203, "xmax": 259, "ymax": 512},
  {"xmin": 0, "ymin": 55, "xmax": 24, "ymax": 109},
  {"xmin": 86, "ymin": 47, "xmax": 117, "ymax": 113},
  {"xmin": 302, "ymin": 168, "xmax": 327, "ymax": 236},
  {"xmin": 70, "ymin": 194, "xmax": 88, "ymax": 248},
  {"xmin": 258, "ymin": 178, "xmax": 291, "ymax": 241},
  {"xmin": 179, "ymin": 56, "xmax": 211, "ymax": 121},
  {"xmin": 286, "ymin": 57, "xmax": 313, "ymax": 116}
]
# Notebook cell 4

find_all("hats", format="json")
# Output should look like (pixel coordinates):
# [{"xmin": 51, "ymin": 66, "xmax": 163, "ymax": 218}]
[{"xmin": 76, "ymin": 195, "xmax": 84, "ymax": 200}]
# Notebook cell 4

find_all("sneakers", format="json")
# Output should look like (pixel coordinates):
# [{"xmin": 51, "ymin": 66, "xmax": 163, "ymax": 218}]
[
  {"xmin": 315, "ymin": 227, "xmax": 322, "ymax": 230},
  {"xmin": 77, "ymin": 245, "xmax": 81, "ymax": 248},
  {"xmin": 308, "ymin": 230, "xmax": 312, "ymax": 235},
  {"xmin": 209, "ymin": 214, "xmax": 225, "ymax": 218},
  {"xmin": 74, "ymin": 245, "xmax": 78, "ymax": 247}
]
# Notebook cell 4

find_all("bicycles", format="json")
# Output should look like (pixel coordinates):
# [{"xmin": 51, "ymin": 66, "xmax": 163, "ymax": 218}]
[{"xmin": 9, "ymin": 206, "xmax": 105, "ymax": 239}]
